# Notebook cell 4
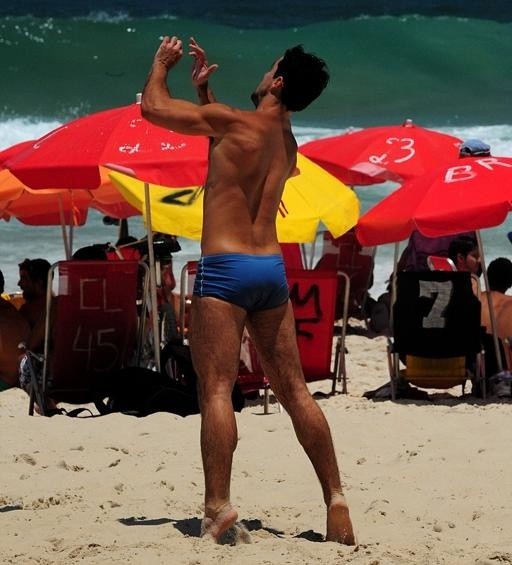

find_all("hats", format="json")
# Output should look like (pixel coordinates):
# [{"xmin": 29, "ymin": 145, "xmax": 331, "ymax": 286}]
[{"xmin": 458, "ymin": 137, "xmax": 491, "ymax": 157}]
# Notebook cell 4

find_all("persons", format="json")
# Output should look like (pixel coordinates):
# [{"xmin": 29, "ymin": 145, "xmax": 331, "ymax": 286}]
[
  {"xmin": 370, "ymin": 217, "xmax": 511, "ymax": 374},
  {"xmin": 72, "ymin": 237, "xmax": 177, "ymax": 295},
  {"xmin": 141, "ymin": 34, "xmax": 359, "ymax": 549},
  {"xmin": 1, "ymin": 272, "xmax": 30, "ymax": 392},
  {"xmin": 0, "ymin": 260, "xmax": 54, "ymax": 373}
]
